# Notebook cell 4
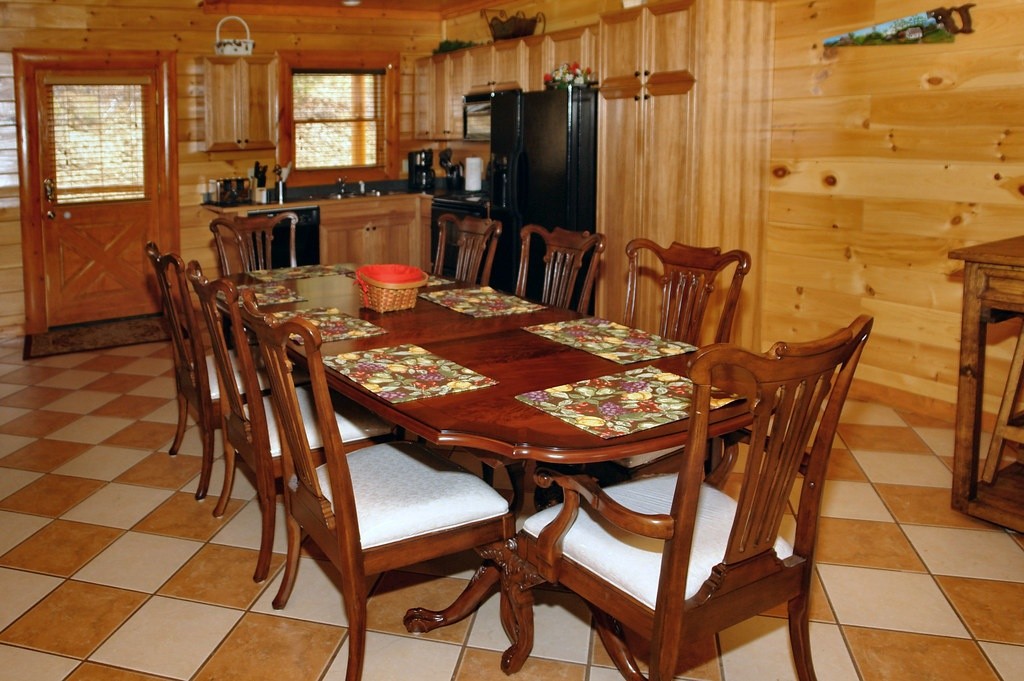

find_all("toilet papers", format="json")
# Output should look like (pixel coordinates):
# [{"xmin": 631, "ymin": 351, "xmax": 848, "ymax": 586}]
[{"xmin": 464, "ymin": 156, "xmax": 484, "ymax": 191}]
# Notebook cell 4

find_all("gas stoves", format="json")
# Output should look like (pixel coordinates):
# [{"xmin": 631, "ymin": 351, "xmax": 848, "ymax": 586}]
[{"xmin": 432, "ymin": 192, "xmax": 494, "ymax": 209}]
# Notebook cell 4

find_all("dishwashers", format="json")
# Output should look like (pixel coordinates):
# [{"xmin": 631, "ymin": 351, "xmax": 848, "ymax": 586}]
[{"xmin": 247, "ymin": 205, "xmax": 321, "ymax": 273}]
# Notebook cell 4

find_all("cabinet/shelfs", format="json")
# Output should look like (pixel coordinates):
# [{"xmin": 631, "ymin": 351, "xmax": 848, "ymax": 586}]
[{"xmin": 204, "ymin": 52, "xmax": 278, "ymax": 151}]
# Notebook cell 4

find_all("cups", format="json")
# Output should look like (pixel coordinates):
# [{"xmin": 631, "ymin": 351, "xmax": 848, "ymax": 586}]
[{"xmin": 274, "ymin": 182, "xmax": 287, "ymax": 204}]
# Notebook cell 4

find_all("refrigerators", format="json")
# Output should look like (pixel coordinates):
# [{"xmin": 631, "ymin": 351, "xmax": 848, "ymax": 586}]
[{"xmin": 489, "ymin": 86, "xmax": 598, "ymax": 317}]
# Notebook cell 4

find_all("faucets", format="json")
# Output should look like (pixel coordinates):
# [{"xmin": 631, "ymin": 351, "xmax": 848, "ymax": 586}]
[{"xmin": 337, "ymin": 174, "xmax": 348, "ymax": 194}]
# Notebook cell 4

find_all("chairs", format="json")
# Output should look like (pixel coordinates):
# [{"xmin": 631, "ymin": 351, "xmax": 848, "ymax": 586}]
[
  {"xmin": 501, "ymin": 316, "xmax": 874, "ymax": 681},
  {"xmin": 210, "ymin": 213, "xmax": 299, "ymax": 350},
  {"xmin": 502, "ymin": 224, "xmax": 605, "ymax": 517},
  {"xmin": 241, "ymin": 288, "xmax": 516, "ymax": 681},
  {"xmin": 145, "ymin": 240, "xmax": 310, "ymax": 500},
  {"xmin": 581, "ymin": 238, "xmax": 751, "ymax": 491},
  {"xmin": 432, "ymin": 213, "xmax": 502, "ymax": 286},
  {"xmin": 186, "ymin": 258, "xmax": 395, "ymax": 584}
]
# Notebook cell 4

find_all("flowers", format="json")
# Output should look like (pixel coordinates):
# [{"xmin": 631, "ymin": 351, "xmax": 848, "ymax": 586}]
[{"xmin": 544, "ymin": 62, "xmax": 592, "ymax": 89}]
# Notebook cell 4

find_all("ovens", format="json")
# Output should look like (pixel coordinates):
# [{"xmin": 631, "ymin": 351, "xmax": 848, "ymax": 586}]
[{"xmin": 431, "ymin": 203, "xmax": 489, "ymax": 287}]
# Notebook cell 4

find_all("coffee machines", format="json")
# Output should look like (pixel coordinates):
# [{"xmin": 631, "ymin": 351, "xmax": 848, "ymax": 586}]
[{"xmin": 407, "ymin": 148, "xmax": 435, "ymax": 194}]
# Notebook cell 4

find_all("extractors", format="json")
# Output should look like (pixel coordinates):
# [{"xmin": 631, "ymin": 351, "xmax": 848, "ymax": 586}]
[{"xmin": 461, "ymin": 87, "xmax": 521, "ymax": 142}]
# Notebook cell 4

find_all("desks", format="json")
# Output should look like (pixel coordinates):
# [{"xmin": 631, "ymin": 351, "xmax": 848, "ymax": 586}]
[
  {"xmin": 212, "ymin": 262, "xmax": 779, "ymax": 588},
  {"xmin": 947, "ymin": 235, "xmax": 1024, "ymax": 534}
]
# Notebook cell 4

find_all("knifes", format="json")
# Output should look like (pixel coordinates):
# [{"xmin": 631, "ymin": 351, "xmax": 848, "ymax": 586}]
[{"xmin": 254, "ymin": 161, "xmax": 268, "ymax": 188}]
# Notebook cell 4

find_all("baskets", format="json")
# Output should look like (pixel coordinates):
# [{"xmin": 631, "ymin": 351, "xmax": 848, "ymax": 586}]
[{"xmin": 357, "ymin": 265, "xmax": 430, "ymax": 312}]
